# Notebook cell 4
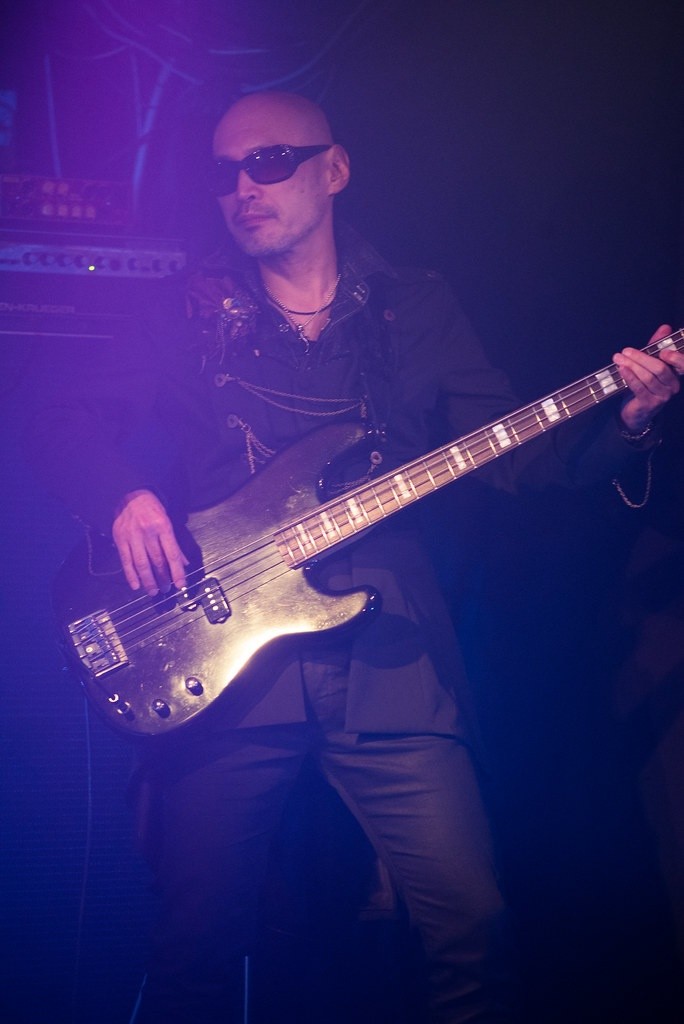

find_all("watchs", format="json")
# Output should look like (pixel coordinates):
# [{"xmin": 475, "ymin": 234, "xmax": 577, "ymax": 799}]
[{"xmin": 617, "ymin": 419, "xmax": 656, "ymax": 442}]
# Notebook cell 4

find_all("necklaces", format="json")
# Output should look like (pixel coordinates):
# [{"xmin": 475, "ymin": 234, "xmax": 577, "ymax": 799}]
[{"xmin": 261, "ymin": 272, "xmax": 341, "ymax": 355}]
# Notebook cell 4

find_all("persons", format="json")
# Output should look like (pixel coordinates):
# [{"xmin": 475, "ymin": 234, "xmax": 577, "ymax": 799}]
[{"xmin": 27, "ymin": 92, "xmax": 684, "ymax": 1024}]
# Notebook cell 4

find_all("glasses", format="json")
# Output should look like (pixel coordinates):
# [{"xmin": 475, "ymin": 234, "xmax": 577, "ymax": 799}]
[{"xmin": 206, "ymin": 144, "xmax": 334, "ymax": 197}]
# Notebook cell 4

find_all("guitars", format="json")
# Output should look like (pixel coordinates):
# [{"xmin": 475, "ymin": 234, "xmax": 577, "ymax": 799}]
[{"xmin": 51, "ymin": 326, "xmax": 684, "ymax": 761}]
